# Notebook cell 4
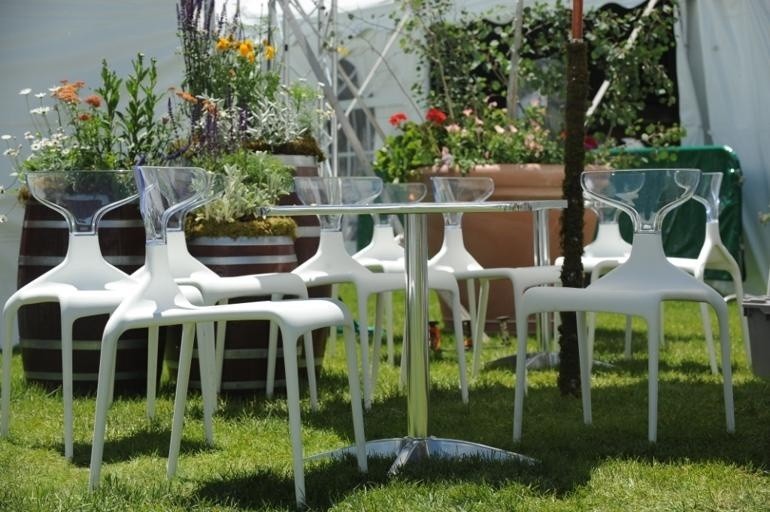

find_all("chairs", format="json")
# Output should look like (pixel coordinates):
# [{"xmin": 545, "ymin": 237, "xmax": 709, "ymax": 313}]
[{"xmin": 1, "ymin": 156, "xmax": 754, "ymax": 508}]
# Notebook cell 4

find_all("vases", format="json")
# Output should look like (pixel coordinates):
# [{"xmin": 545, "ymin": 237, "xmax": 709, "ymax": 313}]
[{"xmin": 404, "ymin": 162, "xmax": 612, "ymax": 337}]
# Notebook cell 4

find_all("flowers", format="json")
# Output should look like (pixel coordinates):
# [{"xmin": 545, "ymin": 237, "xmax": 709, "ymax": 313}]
[
  {"xmin": 372, "ymin": 107, "xmax": 597, "ymax": 185},
  {"xmin": 0, "ymin": 0, "xmax": 335, "ymax": 225}
]
